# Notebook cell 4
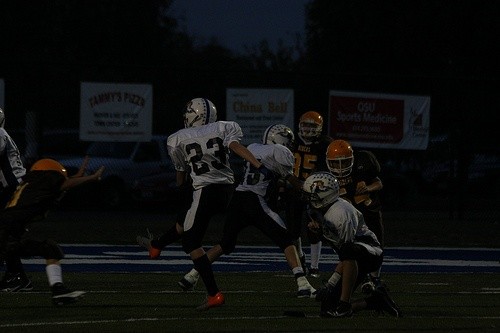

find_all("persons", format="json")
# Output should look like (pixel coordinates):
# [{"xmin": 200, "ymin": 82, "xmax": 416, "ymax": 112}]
[
  {"xmin": 140, "ymin": 98, "xmax": 276, "ymax": 309},
  {"xmin": 177, "ymin": 124, "xmax": 318, "ymax": 298},
  {"xmin": 0, "ymin": 159, "xmax": 104, "ymax": 306},
  {"xmin": 0, "ymin": 107, "xmax": 35, "ymax": 293},
  {"xmin": 285, "ymin": 111, "xmax": 385, "ymax": 279},
  {"xmin": 302, "ymin": 171, "xmax": 401, "ymax": 318}
]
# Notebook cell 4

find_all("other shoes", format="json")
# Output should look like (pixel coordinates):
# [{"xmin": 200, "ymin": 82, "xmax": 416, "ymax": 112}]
[
  {"xmin": 310, "ymin": 268, "xmax": 321, "ymax": 278},
  {"xmin": 3, "ymin": 271, "xmax": 33, "ymax": 292},
  {"xmin": 0, "ymin": 271, "xmax": 17, "ymax": 291},
  {"xmin": 297, "ymin": 284, "xmax": 318, "ymax": 299},
  {"xmin": 51, "ymin": 286, "xmax": 88, "ymax": 306},
  {"xmin": 195, "ymin": 291, "xmax": 225, "ymax": 312},
  {"xmin": 376, "ymin": 284, "xmax": 402, "ymax": 319},
  {"xmin": 327, "ymin": 300, "xmax": 353, "ymax": 318},
  {"xmin": 360, "ymin": 279, "xmax": 376, "ymax": 296},
  {"xmin": 136, "ymin": 228, "xmax": 161, "ymax": 259},
  {"xmin": 178, "ymin": 275, "xmax": 199, "ymax": 292}
]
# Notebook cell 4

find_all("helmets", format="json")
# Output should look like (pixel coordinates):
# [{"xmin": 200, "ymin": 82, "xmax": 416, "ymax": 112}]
[
  {"xmin": 0, "ymin": 109, "xmax": 5, "ymax": 128},
  {"xmin": 186, "ymin": 98, "xmax": 217, "ymax": 127},
  {"xmin": 303, "ymin": 171, "xmax": 340, "ymax": 208},
  {"xmin": 327, "ymin": 140, "xmax": 353, "ymax": 160},
  {"xmin": 299, "ymin": 111, "xmax": 323, "ymax": 127},
  {"xmin": 30, "ymin": 158, "xmax": 68, "ymax": 177},
  {"xmin": 262, "ymin": 123, "xmax": 294, "ymax": 146}
]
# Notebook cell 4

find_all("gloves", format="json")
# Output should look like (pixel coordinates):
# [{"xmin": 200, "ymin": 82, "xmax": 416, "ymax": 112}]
[
  {"xmin": 312, "ymin": 283, "xmax": 336, "ymax": 303},
  {"xmin": 257, "ymin": 164, "xmax": 274, "ymax": 181}
]
